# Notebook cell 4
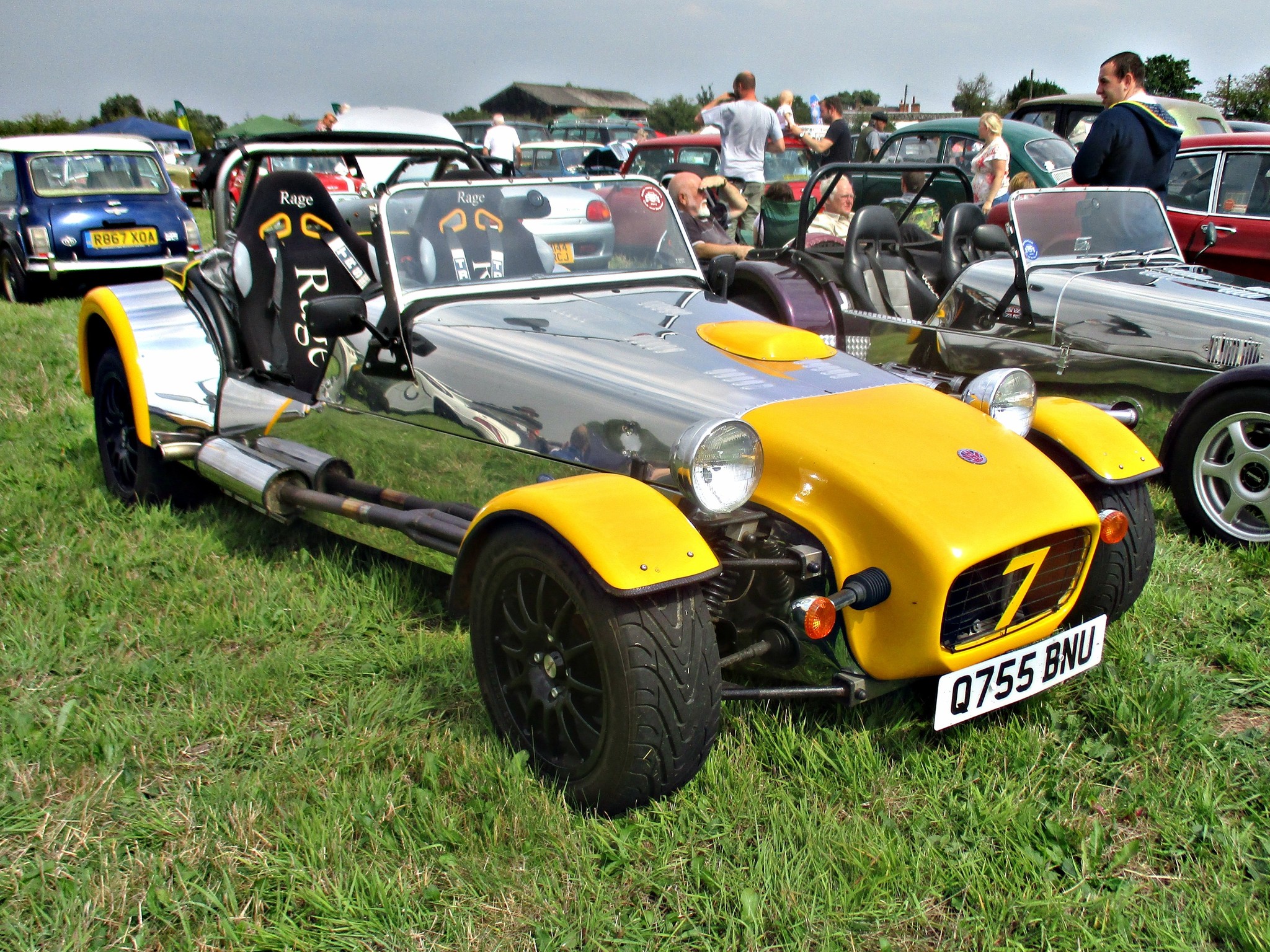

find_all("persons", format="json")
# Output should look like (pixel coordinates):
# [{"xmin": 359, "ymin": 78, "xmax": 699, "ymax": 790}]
[
  {"xmin": 482, "ymin": 112, "xmax": 523, "ymax": 176},
  {"xmin": 315, "ymin": 114, "xmax": 337, "ymax": 132},
  {"xmin": 1071, "ymin": 51, "xmax": 1184, "ymax": 255},
  {"xmin": 970, "ymin": 112, "xmax": 1011, "ymax": 222},
  {"xmin": 549, "ymin": 425, "xmax": 589, "ymax": 463},
  {"xmin": 163, "ymin": 151, "xmax": 188, "ymax": 166},
  {"xmin": 1008, "ymin": 171, "xmax": 1037, "ymax": 204},
  {"xmin": 622, "ymin": 71, "xmax": 986, "ymax": 279}
]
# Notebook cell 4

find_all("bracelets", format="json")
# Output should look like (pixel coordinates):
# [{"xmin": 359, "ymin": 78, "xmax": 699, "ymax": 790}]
[{"xmin": 720, "ymin": 176, "xmax": 727, "ymax": 190}]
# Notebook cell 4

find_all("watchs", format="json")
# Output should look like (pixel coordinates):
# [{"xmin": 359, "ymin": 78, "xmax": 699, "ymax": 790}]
[{"xmin": 797, "ymin": 129, "xmax": 806, "ymax": 139}]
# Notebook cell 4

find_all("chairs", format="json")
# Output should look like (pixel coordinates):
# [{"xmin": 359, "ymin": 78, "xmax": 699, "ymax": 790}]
[
  {"xmin": 410, "ymin": 168, "xmax": 555, "ymax": 294},
  {"xmin": 944, "ymin": 202, "xmax": 995, "ymax": 294},
  {"xmin": 3, "ymin": 167, "xmax": 50, "ymax": 208},
  {"xmin": 845, "ymin": 204, "xmax": 941, "ymax": 321},
  {"xmin": 90, "ymin": 171, "xmax": 135, "ymax": 193},
  {"xmin": 235, "ymin": 172, "xmax": 372, "ymax": 390}
]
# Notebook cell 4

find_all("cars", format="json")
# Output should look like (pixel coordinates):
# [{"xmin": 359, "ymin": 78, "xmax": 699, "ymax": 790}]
[
  {"xmin": 89, "ymin": 91, "xmax": 1270, "ymax": 283},
  {"xmin": 0, "ymin": 134, "xmax": 202, "ymax": 307},
  {"xmin": 704, "ymin": 162, "xmax": 1269, "ymax": 553},
  {"xmin": 74, "ymin": 130, "xmax": 1163, "ymax": 824}
]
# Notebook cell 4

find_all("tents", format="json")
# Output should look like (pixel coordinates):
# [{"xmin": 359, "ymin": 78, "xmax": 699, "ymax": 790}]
[
  {"xmin": 529, "ymin": 113, "xmax": 648, "ymax": 145},
  {"xmin": 55, "ymin": 116, "xmax": 193, "ymax": 176},
  {"xmin": 215, "ymin": 114, "xmax": 308, "ymax": 136}
]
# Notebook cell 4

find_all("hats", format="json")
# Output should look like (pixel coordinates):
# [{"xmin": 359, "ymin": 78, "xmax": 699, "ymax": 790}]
[{"xmin": 871, "ymin": 112, "xmax": 888, "ymax": 122}]
